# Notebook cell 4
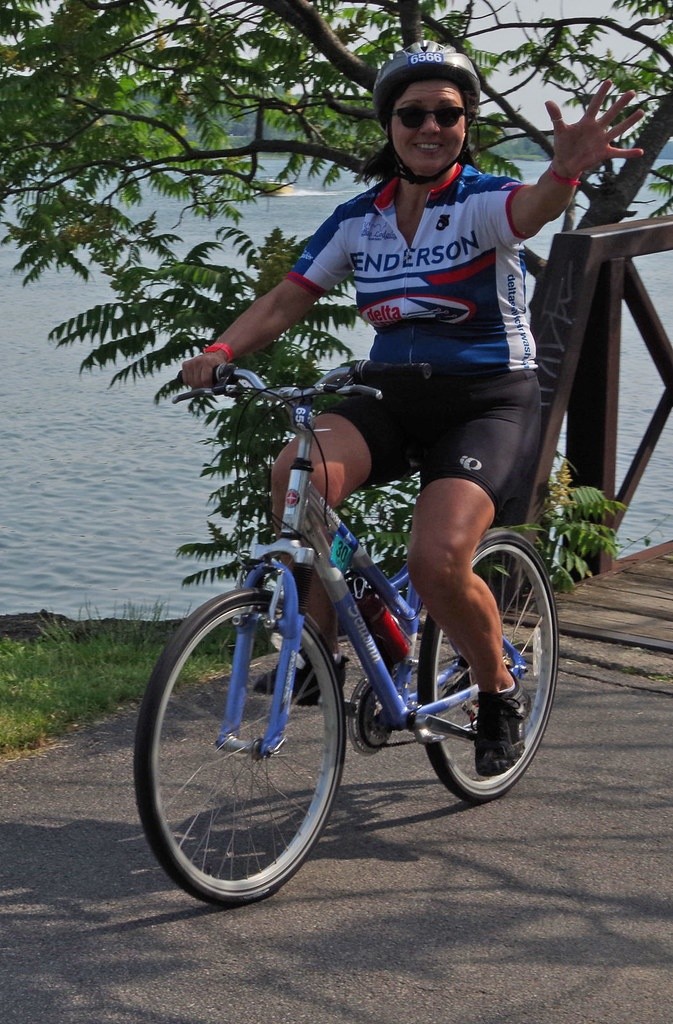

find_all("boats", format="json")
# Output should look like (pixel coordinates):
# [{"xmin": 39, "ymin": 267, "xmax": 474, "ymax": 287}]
[{"xmin": 254, "ymin": 176, "xmax": 294, "ymax": 196}]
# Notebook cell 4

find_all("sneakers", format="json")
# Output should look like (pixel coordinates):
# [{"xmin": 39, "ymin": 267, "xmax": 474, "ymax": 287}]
[
  {"xmin": 471, "ymin": 670, "xmax": 532, "ymax": 778},
  {"xmin": 253, "ymin": 646, "xmax": 350, "ymax": 708}
]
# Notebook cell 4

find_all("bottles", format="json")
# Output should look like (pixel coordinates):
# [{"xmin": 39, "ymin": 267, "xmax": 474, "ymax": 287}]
[{"xmin": 358, "ymin": 597, "xmax": 409, "ymax": 666}]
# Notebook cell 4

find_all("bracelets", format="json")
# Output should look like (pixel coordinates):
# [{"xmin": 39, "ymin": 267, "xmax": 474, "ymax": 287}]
[
  {"xmin": 547, "ymin": 162, "xmax": 582, "ymax": 186},
  {"xmin": 206, "ymin": 343, "xmax": 234, "ymax": 363}
]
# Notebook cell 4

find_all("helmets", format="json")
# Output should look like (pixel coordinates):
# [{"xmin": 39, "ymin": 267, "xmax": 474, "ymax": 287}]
[{"xmin": 372, "ymin": 40, "xmax": 481, "ymax": 131}]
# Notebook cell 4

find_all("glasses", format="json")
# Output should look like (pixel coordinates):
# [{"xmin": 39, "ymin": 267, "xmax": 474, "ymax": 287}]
[{"xmin": 388, "ymin": 105, "xmax": 468, "ymax": 129}]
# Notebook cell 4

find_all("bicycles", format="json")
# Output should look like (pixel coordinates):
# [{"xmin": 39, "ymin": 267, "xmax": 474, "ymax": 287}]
[{"xmin": 130, "ymin": 358, "xmax": 561, "ymax": 909}]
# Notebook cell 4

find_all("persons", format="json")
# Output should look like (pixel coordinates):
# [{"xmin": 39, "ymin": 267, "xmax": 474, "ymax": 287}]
[{"xmin": 179, "ymin": 38, "xmax": 648, "ymax": 779}]
